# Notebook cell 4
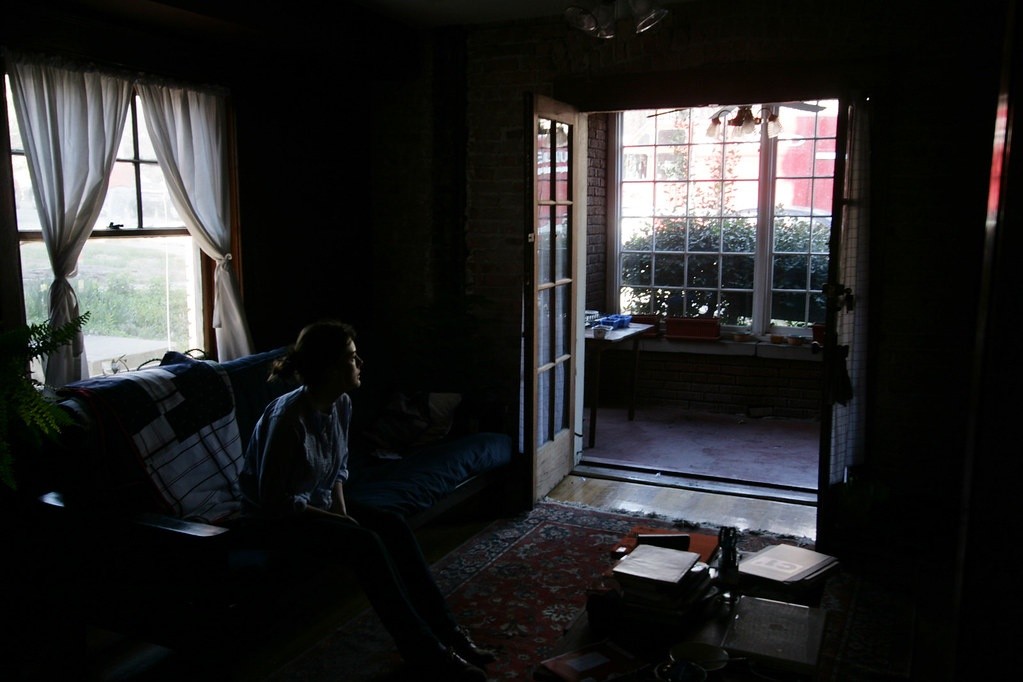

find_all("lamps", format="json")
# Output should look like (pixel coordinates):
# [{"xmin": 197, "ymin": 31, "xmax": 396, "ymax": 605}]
[{"xmin": 564, "ymin": 0, "xmax": 669, "ymax": 40}]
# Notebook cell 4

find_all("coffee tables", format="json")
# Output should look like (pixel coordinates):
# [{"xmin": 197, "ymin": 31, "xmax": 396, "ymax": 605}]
[{"xmin": 530, "ymin": 563, "xmax": 831, "ymax": 681}]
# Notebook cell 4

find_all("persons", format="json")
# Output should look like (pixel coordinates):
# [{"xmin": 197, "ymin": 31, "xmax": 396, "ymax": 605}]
[{"xmin": 239, "ymin": 324, "xmax": 494, "ymax": 682}]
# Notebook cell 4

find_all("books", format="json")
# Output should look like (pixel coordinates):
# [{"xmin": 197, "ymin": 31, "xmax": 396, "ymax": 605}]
[
  {"xmin": 613, "ymin": 544, "xmax": 700, "ymax": 583},
  {"xmin": 635, "ymin": 534, "xmax": 690, "ymax": 550},
  {"xmin": 539, "ymin": 639, "xmax": 650, "ymax": 682}
]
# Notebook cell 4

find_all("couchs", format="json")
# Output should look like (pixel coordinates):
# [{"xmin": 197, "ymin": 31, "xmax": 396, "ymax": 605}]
[{"xmin": 49, "ymin": 344, "xmax": 514, "ymax": 649}]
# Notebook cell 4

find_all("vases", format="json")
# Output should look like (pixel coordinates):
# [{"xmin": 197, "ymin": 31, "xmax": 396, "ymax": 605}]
[
  {"xmin": 770, "ymin": 336, "xmax": 783, "ymax": 344},
  {"xmin": 786, "ymin": 334, "xmax": 806, "ymax": 344},
  {"xmin": 664, "ymin": 317, "xmax": 722, "ymax": 341},
  {"xmin": 630, "ymin": 314, "xmax": 660, "ymax": 337}
]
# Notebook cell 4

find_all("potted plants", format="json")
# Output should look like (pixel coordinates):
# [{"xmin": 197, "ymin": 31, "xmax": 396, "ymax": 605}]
[{"xmin": 732, "ymin": 331, "xmax": 747, "ymax": 341}]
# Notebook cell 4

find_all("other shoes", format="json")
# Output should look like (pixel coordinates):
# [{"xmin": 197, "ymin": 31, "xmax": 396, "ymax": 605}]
[
  {"xmin": 441, "ymin": 624, "xmax": 496, "ymax": 665},
  {"xmin": 401, "ymin": 642, "xmax": 486, "ymax": 682}
]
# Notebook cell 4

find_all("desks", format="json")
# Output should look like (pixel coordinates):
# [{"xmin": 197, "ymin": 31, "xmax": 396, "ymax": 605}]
[{"xmin": 585, "ymin": 322, "xmax": 656, "ymax": 448}]
